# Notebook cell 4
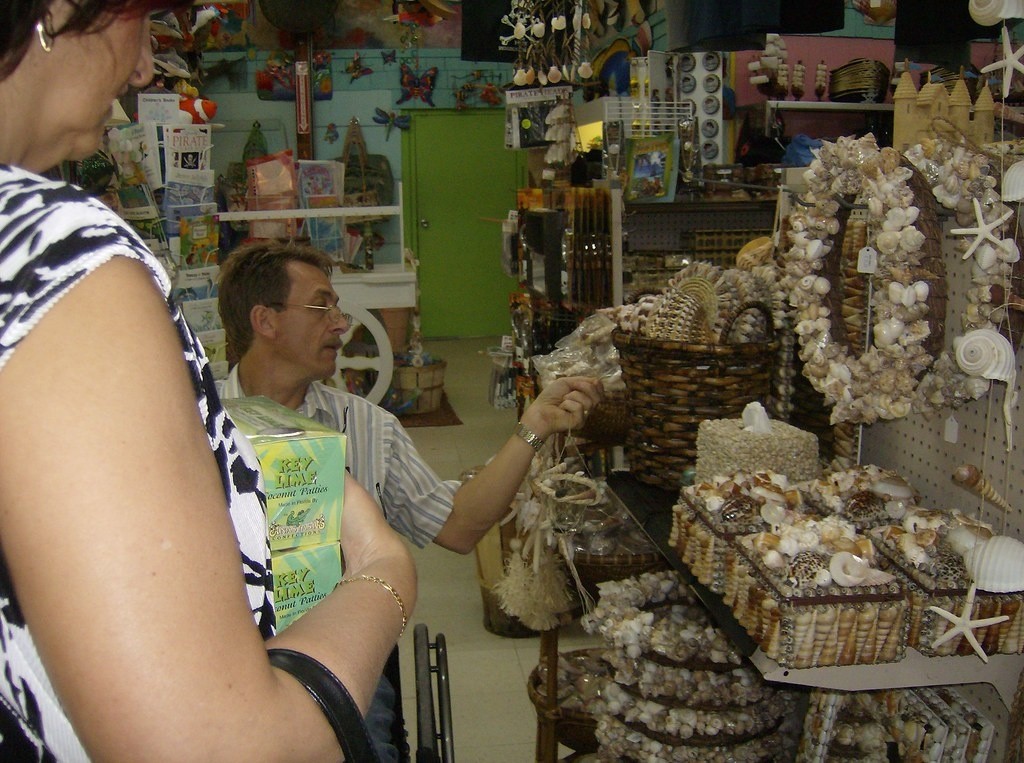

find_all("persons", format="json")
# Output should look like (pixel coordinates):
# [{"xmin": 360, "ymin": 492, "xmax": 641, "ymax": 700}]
[
  {"xmin": 197, "ymin": 246, "xmax": 606, "ymax": 763},
  {"xmin": 0, "ymin": 0, "xmax": 416, "ymax": 763}
]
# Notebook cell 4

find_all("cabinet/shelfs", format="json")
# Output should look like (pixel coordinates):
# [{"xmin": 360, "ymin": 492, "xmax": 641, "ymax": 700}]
[{"xmin": 49, "ymin": 0, "xmax": 1024, "ymax": 763}]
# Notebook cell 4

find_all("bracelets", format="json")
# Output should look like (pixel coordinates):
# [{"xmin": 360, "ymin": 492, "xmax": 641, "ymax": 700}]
[
  {"xmin": 514, "ymin": 421, "xmax": 544, "ymax": 452},
  {"xmin": 328, "ymin": 574, "xmax": 408, "ymax": 643}
]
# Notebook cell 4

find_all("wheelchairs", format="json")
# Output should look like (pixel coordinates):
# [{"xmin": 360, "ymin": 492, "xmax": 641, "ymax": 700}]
[{"xmin": 407, "ymin": 621, "xmax": 456, "ymax": 763}]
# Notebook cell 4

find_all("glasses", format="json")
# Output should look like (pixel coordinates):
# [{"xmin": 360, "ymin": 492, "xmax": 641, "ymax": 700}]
[{"xmin": 265, "ymin": 301, "xmax": 353, "ymax": 331}]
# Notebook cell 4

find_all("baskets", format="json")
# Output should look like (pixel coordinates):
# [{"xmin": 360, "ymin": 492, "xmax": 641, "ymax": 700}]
[
  {"xmin": 611, "ymin": 288, "xmax": 781, "ymax": 492},
  {"xmin": 477, "ymin": 573, "xmax": 542, "ymax": 638},
  {"xmin": 558, "ymin": 552, "xmax": 667, "ymax": 606},
  {"xmin": 527, "ymin": 647, "xmax": 607, "ymax": 753},
  {"xmin": 563, "ymin": 391, "xmax": 628, "ymax": 446}
]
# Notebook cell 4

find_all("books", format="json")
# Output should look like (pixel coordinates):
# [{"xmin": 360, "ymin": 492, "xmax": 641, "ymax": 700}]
[
  {"xmin": 623, "ymin": 131, "xmax": 680, "ymax": 205},
  {"xmin": 108, "ymin": 93, "xmax": 229, "ymax": 384},
  {"xmin": 246, "ymin": 153, "xmax": 346, "ymax": 235}
]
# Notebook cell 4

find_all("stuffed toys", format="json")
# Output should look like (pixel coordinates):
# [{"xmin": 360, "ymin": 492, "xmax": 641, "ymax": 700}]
[{"xmin": 134, "ymin": 94, "xmax": 217, "ymax": 124}]
